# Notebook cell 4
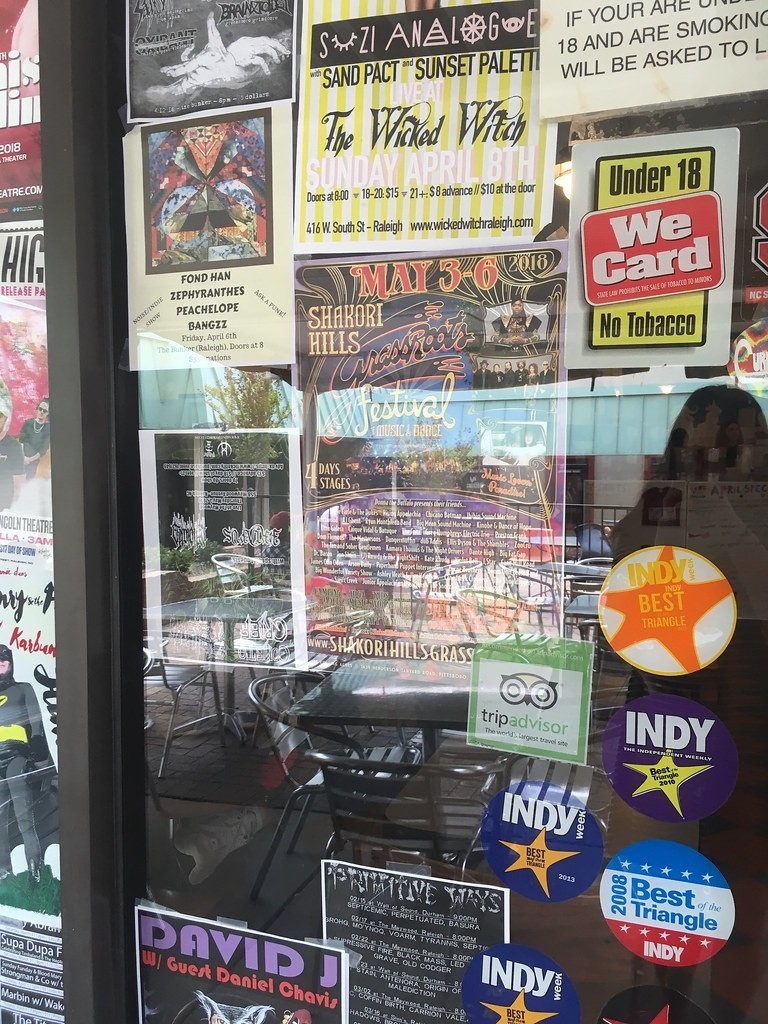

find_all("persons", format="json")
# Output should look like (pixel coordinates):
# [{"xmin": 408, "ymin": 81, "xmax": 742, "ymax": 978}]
[
  {"xmin": 0, "ymin": 402, "xmax": 26, "ymax": 517},
  {"xmin": 608, "ymin": 384, "xmax": 767, "ymax": 1024},
  {"xmin": 474, "ymin": 359, "xmax": 555, "ymax": 409},
  {"xmin": 19, "ymin": 398, "xmax": 50, "ymax": 486},
  {"xmin": 1, "ymin": 644, "xmax": 58, "ymax": 886},
  {"xmin": 346, "ymin": 426, "xmax": 546, "ymax": 486},
  {"xmin": 490, "ymin": 297, "xmax": 542, "ymax": 346}
]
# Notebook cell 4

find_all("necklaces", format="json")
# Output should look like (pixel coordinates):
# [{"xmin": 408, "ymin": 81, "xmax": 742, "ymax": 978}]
[{"xmin": 33, "ymin": 416, "xmax": 44, "ymax": 434}]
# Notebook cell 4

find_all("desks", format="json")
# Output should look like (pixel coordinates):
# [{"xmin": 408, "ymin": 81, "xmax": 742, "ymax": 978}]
[
  {"xmin": 143, "ymin": 597, "xmax": 316, "ymax": 748},
  {"xmin": 281, "ymin": 656, "xmax": 556, "ymax": 875},
  {"xmin": 565, "ymin": 595, "xmax": 600, "ymax": 616},
  {"xmin": 536, "ymin": 560, "xmax": 611, "ymax": 577}
]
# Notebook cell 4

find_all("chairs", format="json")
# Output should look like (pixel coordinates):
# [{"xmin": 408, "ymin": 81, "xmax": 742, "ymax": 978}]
[{"xmin": 133, "ymin": 551, "xmax": 628, "ymax": 1024}]
